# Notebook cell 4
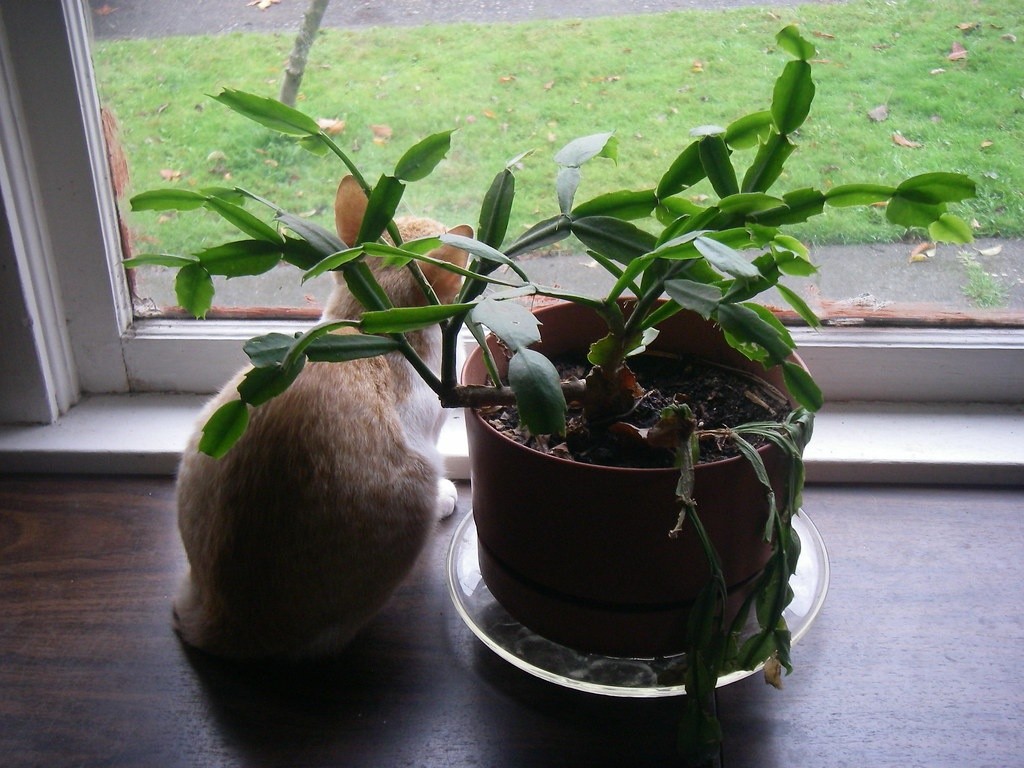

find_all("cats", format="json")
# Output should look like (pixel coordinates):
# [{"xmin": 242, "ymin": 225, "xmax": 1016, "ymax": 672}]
[{"xmin": 168, "ymin": 174, "xmax": 473, "ymax": 671}]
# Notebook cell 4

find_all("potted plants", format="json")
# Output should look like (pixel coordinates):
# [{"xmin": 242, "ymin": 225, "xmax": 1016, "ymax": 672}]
[{"xmin": 119, "ymin": 23, "xmax": 976, "ymax": 768}]
